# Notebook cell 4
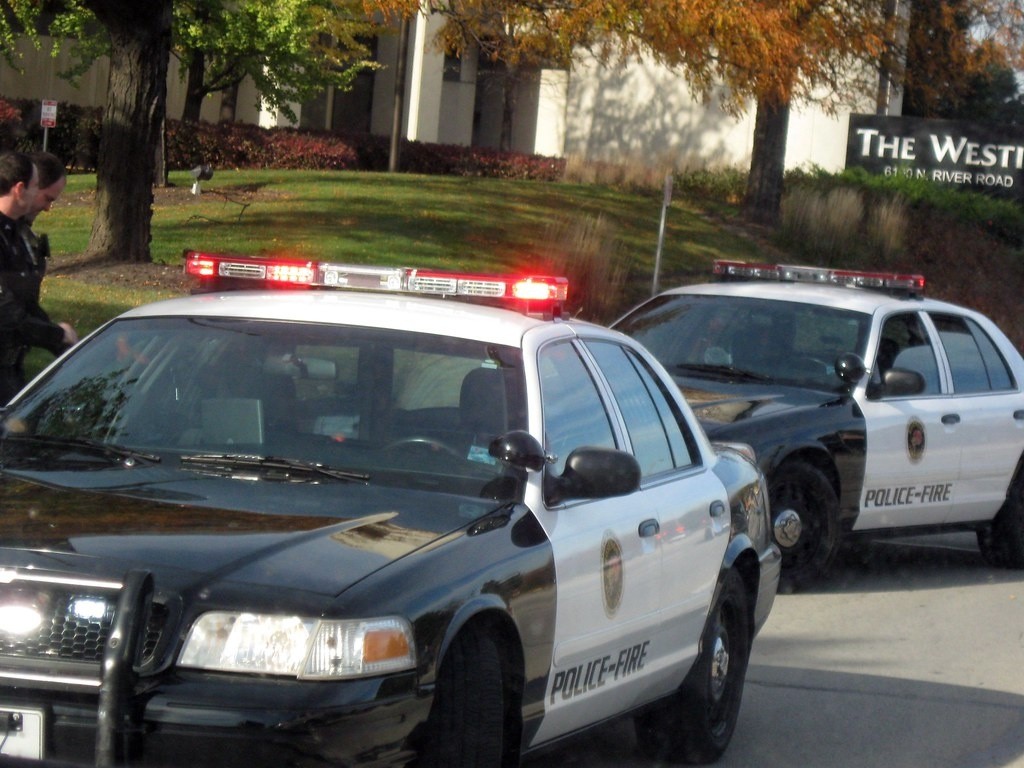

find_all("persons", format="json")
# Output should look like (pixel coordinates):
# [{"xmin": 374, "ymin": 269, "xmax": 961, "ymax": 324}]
[{"xmin": 0, "ymin": 151, "xmax": 78, "ymax": 408}]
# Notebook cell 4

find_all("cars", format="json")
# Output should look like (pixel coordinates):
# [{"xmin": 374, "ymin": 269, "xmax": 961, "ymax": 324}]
[
  {"xmin": 588, "ymin": 256, "xmax": 1023, "ymax": 590},
  {"xmin": 2, "ymin": 249, "xmax": 782, "ymax": 768}
]
